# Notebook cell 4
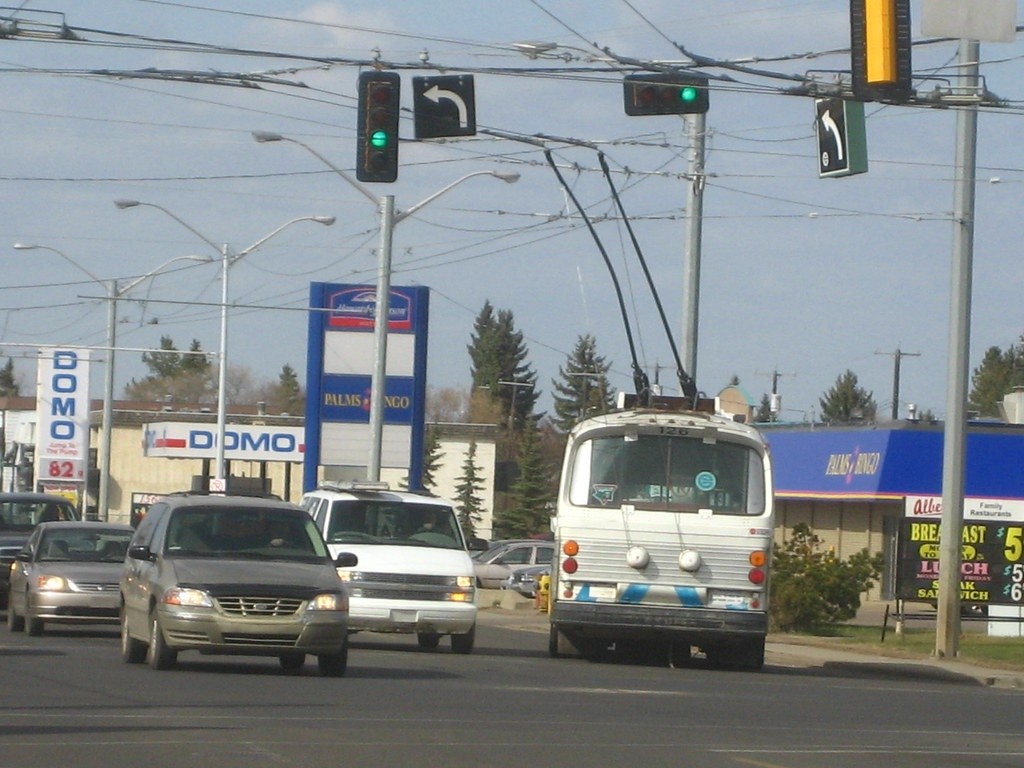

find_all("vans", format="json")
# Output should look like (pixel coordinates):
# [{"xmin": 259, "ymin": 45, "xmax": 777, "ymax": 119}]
[{"xmin": 296, "ymin": 483, "xmax": 490, "ymax": 651}]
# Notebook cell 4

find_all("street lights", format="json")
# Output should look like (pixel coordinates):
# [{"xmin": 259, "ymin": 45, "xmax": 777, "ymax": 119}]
[
  {"xmin": 250, "ymin": 125, "xmax": 519, "ymax": 532},
  {"xmin": 112, "ymin": 196, "xmax": 339, "ymax": 536},
  {"xmin": 15, "ymin": 239, "xmax": 212, "ymax": 552}
]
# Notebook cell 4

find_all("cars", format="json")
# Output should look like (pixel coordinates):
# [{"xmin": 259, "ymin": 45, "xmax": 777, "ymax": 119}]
[
  {"xmin": 466, "ymin": 537, "xmax": 554, "ymax": 597},
  {"xmin": 5, "ymin": 520, "xmax": 135, "ymax": 638}
]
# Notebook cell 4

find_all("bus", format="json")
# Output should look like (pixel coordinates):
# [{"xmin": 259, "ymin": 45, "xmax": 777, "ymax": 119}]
[{"xmin": 540, "ymin": 142, "xmax": 777, "ymax": 675}]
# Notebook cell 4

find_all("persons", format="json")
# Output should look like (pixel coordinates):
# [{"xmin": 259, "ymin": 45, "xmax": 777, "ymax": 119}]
[
  {"xmin": 263, "ymin": 519, "xmax": 303, "ymax": 550},
  {"xmin": 415, "ymin": 512, "xmax": 451, "ymax": 537}
]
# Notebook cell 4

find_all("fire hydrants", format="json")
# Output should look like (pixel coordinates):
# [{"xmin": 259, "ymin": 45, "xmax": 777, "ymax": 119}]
[{"xmin": 533, "ymin": 570, "xmax": 552, "ymax": 613}]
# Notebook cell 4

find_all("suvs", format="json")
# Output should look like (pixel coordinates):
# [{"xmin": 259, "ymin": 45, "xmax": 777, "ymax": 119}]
[
  {"xmin": 0, "ymin": 490, "xmax": 82, "ymax": 613},
  {"xmin": 116, "ymin": 489, "xmax": 359, "ymax": 677}
]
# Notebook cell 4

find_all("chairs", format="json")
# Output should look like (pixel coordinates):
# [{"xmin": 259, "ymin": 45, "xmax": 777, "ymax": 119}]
[
  {"xmin": 48, "ymin": 540, "xmax": 69, "ymax": 558},
  {"xmin": 101, "ymin": 542, "xmax": 122, "ymax": 557}
]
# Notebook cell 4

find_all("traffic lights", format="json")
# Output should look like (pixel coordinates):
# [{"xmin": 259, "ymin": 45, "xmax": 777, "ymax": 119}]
[
  {"xmin": 623, "ymin": 72, "xmax": 710, "ymax": 116},
  {"xmin": 355, "ymin": 70, "xmax": 401, "ymax": 184}
]
